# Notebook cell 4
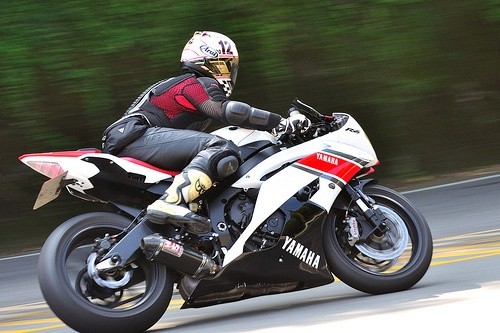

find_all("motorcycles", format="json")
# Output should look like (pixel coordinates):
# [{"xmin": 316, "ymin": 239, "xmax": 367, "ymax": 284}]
[{"xmin": 16, "ymin": 92, "xmax": 433, "ymax": 333}]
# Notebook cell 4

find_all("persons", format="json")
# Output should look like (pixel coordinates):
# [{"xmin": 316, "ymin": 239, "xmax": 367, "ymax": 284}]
[{"xmin": 103, "ymin": 30, "xmax": 310, "ymax": 237}]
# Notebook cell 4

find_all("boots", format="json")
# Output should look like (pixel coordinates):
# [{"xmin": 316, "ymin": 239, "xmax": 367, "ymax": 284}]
[{"xmin": 145, "ymin": 169, "xmax": 212, "ymax": 235}]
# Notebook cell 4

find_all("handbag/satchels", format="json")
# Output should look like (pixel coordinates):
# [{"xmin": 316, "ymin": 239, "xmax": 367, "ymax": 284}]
[{"xmin": 97, "ymin": 113, "xmax": 149, "ymax": 156}]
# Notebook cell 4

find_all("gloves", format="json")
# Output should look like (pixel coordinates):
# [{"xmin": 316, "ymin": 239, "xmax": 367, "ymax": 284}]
[{"xmin": 276, "ymin": 111, "xmax": 314, "ymax": 135}]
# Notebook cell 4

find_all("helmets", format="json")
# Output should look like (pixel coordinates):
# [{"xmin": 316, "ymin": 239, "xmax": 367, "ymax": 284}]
[{"xmin": 180, "ymin": 28, "xmax": 242, "ymax": 98}]
